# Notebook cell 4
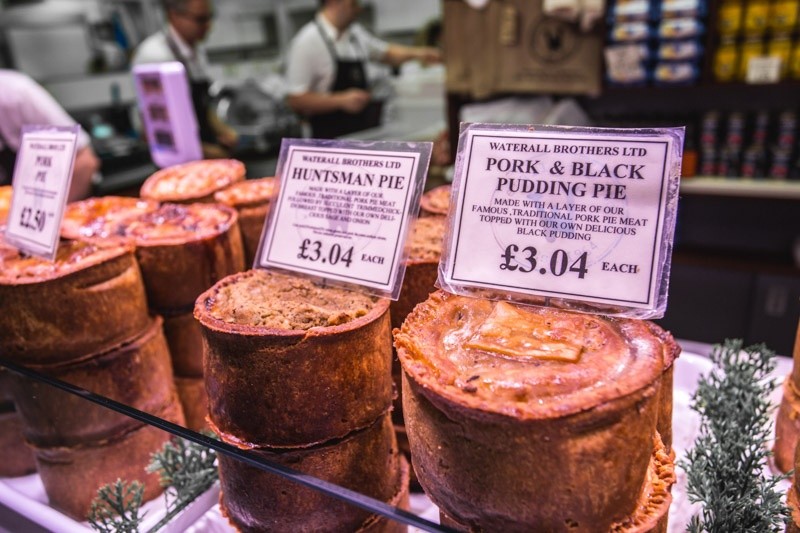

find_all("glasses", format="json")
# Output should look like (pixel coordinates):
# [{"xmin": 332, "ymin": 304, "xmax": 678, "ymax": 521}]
[{"xmin": 178, "ymin": 12, "xmax": 213, "ymax": 23}]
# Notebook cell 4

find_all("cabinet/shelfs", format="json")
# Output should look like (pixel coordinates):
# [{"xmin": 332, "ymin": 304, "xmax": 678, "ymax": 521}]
[
  {"xmin": 600, "ymin": 0, "xmax": 800, "ymax": 358},
  {"xmin": 0, "ymin": 0, "xmax": 382, "ymax": 197}
]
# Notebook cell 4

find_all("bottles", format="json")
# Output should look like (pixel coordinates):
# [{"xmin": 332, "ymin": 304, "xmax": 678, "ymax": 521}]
[
  {"xmin": 679, "ymin": 140, "xmax": 696, "ymax": 177},
  {"xmin": 700, "ymin": 112, "xmax": 795, "ymax": 177}
]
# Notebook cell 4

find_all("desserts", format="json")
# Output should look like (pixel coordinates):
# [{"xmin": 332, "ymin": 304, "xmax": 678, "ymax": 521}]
[
  {"xmin": 776, "ymin": 314, "xmax": 800, "ymax": 533},
  {"xmin": 0, "ymin": 160, "xmax": 681, "ymax": 532}
]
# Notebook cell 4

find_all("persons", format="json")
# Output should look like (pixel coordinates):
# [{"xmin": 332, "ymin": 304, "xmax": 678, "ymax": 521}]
[
  {"xmin": 0, "ymin": 69, "xmax": 100, "ymax": 204},
  {"xmin": 131, "ymin": 1, "xmax": 236, "ymax": 165},
  {"xmin": 285, "ymin": 1, "xmax": 444, "ymax": 145}
]
